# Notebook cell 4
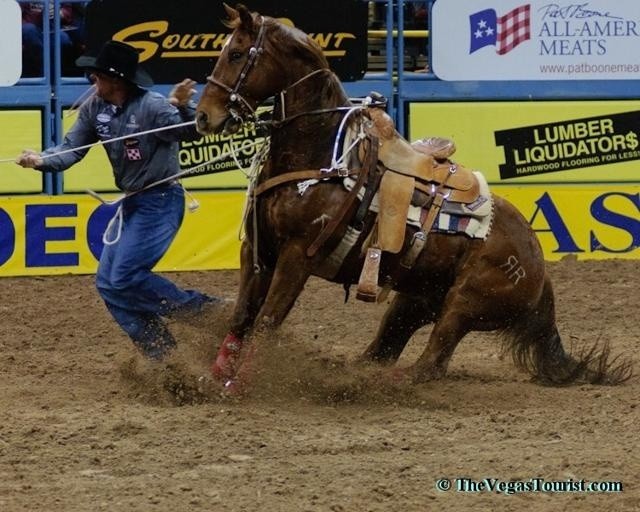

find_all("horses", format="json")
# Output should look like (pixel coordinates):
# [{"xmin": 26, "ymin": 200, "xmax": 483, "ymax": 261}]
[{"xmin": 195, "ymin": 2, "xmax": 639, "ymax": 401}]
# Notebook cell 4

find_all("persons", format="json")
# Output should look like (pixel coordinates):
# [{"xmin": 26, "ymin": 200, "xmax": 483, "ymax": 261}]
[
  {"xmin": 407, "ymin": 0, "xmax": 430, "ymax": 74},
  {"xmin": 22, "ymin": 0, "xmax": 84, "ymax": 77},
  {"xmin": 16, "ymin": 41, "xmax": 236, "ymax": 380}
]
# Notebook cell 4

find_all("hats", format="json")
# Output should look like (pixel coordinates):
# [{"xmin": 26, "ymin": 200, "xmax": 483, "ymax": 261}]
[{"xmin": 75, "ymin": 41, "xmax": 155, "ymax": 88}]
